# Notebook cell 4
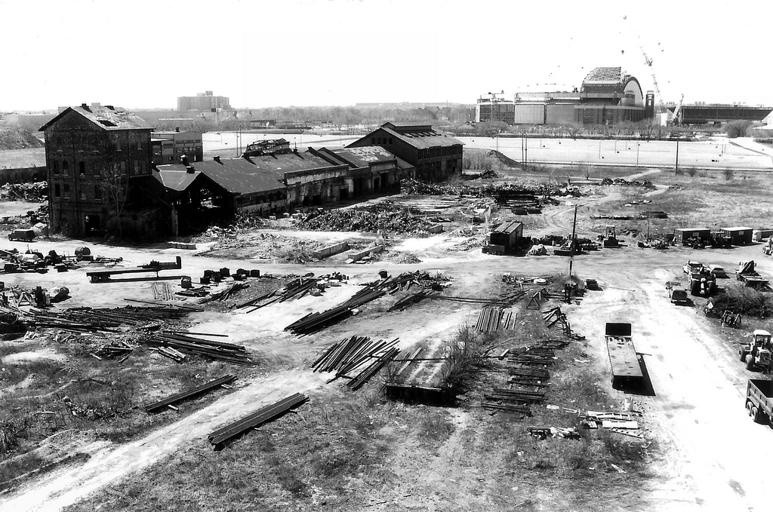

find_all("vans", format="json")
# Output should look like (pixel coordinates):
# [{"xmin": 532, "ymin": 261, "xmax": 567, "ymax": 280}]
[{"xmin": 8, "ymin": 229, "xmax": 36, "ymax": 242}]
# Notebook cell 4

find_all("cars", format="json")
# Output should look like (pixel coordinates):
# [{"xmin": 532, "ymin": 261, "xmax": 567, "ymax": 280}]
[
  {"xmin": 682, "ymin": 262, "xmax": 727, "ymax": 294},
  {"xmin": 585, "ymin": 279, "xmax": 598, "ymax": 289}
]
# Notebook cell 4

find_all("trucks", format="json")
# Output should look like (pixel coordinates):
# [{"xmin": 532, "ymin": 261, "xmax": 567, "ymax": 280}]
[
  {"xmin": 605, "ymin": 322, "xmax": 645, "ymax": 387},
  {"xmin": 667, "ymin": 280, "xmax": 686, "ymax": 304},
  {"xmin": 482, "ymin": 220, "xmax": 523, "ymax": 255}
]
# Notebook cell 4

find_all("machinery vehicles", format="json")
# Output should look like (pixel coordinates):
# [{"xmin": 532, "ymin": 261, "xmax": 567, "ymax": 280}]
[
  {"xmin": 738, "ymin": 329, "xmax": 773, "ymax": 427},
  {"xmin": 603, "ymin": 224, "xmax": 620, "ymax": 248}
]
